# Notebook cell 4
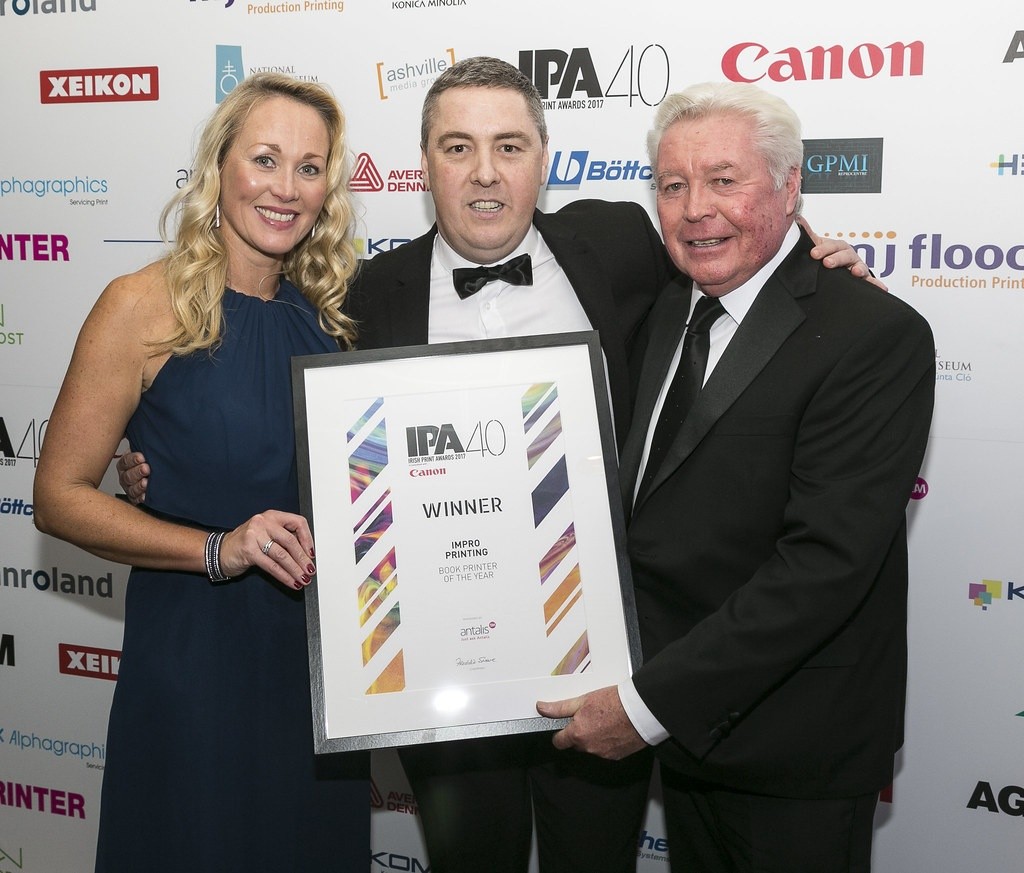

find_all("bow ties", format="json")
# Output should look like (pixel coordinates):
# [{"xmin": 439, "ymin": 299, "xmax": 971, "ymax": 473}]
[{"xmin": 452, "ymin": 253, "xmax": 533, "ymax": 300}]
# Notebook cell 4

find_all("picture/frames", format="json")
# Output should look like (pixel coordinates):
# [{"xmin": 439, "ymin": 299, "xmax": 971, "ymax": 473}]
[{"xmin": 291, "ymin": 328, "xmax": 645, "ymax": 757}]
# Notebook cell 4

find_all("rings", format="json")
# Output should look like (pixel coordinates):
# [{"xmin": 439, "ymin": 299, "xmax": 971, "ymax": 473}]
[{"xmin": 261, "ymin": 540, "xmax": 274, "ymax": 554}]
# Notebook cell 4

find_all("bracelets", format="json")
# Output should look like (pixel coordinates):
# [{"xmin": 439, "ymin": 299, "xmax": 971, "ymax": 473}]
[{"xmin": 205, "ymin": 532, "xmax": 232, "ymax": 586}]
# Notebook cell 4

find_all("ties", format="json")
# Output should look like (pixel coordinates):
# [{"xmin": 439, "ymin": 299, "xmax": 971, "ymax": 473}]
[{"xmin": 634, "ymin": 296, "xmax": 726, "ymax": 514}]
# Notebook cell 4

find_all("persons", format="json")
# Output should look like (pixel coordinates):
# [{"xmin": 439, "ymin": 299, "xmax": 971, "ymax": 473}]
[
  {"xmin": 537, "ymin": 79, "xmax": 938, "ymax": 872},
  {"xmin": 34, "ymin": 69, "xmax": 373, "ymax": 872},
  {"xmin": 116, "ymin": 56, "xmax": 888, "ymax": 873}
]
